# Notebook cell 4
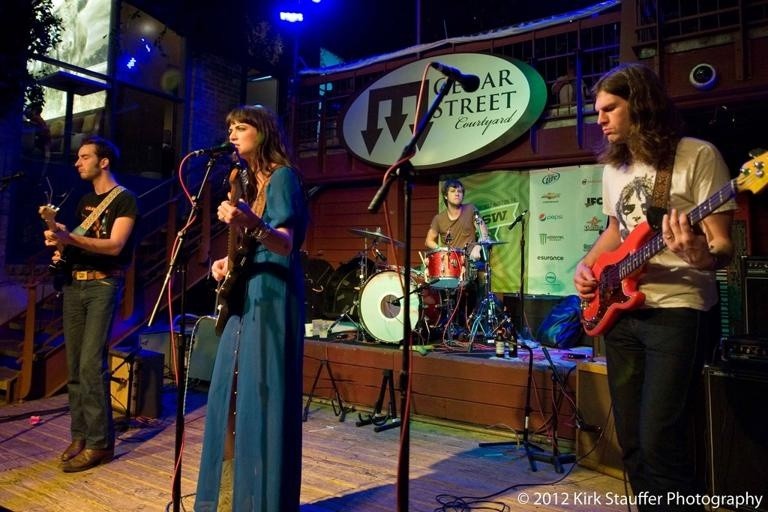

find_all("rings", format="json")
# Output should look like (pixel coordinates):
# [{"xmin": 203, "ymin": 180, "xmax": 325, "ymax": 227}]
[{"xmin": 663, "ymin": 233, "xmax": 673, "ymax": 241}]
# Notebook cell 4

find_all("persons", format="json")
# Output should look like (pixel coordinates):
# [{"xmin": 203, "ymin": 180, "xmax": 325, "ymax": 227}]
[
  {"xmin": 194, "ymin": 104, "xmax": 307, "ymax": 511},
  {"xmin": 22, "ymin": 98, "xmax": 53, "ymax": 161},
  {"xmin": 42, "ymin": 136, "xmax": 139, "ymax": 473},
  {"xmin": 573, "ymin": 61, "xmax": 739, "ymax": 512},
  {"xmin": 423, "ymin": 179, "xmax": 488, "ymax": 324}
]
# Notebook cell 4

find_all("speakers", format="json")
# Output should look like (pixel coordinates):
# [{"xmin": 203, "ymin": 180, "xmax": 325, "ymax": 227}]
[{"xmin": 106, "ymin": 346, "xmax": 164, "ymax": 417}]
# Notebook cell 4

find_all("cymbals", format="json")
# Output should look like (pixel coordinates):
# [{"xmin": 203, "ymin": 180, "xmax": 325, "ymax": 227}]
[
  {"xmin": 347, "ymin": 225, "xmax": 404, "ymax": 247},
  {"xmin": 471, "ymin": 239, "xmax": 508, "ymax": 245}
]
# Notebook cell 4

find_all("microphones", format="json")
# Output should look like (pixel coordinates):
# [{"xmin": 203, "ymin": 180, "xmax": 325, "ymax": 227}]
[
  {"xmin": 430, "ymin": 61, "xmax": 480, "ymax": 92},
  {"xmin": 109, "ymin": 375, "xmax": 126, "ymax": 385},
  {"xmin": 0, "ymin": 171, "xmax": 24, "ymax": 182},
  {"xmin": 509, "ymin": 208, "xmax": 528, "ymax": 229},
  {"xmin": 194, "ymin": 141, "xmax": 235, "ymax": 158}
]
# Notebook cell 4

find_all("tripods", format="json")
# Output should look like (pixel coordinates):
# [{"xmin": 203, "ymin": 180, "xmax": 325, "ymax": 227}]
[
  {"xmin": 109, "ymin": 347, "xmax": 155, "ymax": 435},
  {"xmin": 329, "ymin": 219, "xmax": 534, "ymax": 352},
  {"xmin": 480, "ymin": 344, "xmax": 601, "ymax": 474}
]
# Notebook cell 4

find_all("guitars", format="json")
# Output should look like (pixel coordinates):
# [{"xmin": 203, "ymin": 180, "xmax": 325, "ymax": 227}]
[
  {"xmin": 579, "ymin": 154, "xmax": 767, "ymax": 337},
  {"xmin": 38, "ymin": 203, "xmax": 72, "ymax": 292},
  {"xmin": 213, "ymin": 169, "xmax": 248, "ymax": 333}
]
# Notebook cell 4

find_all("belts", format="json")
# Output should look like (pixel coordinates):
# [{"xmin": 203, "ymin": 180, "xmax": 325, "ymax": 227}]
[{"xmin": 71, "ymin": 268, "xmax": 110, "ymax": 282}]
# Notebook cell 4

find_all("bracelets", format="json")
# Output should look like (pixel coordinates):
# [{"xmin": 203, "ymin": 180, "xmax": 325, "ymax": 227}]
[{"xmin": 247, "ymin": 221, "xmax": 272, "ymax": 243}]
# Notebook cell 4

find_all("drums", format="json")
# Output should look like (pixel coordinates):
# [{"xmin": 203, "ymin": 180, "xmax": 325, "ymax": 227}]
[
  {"xmin": 355, "ymin": 265, "xmax": 443, "ymax": 347},
  {"xmin": 425, "ymin": 246, "xmax": 468, "ymax": 292}
]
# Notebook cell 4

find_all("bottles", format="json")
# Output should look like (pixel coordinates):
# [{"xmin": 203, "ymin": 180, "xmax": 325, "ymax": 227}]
[
  {"xmin": 508, "ymin": 324, "xmax": 518, "ymax": 358},
  {"xmin": 494, "ymin": 327, "xmax": 504, "ymax": 358},
  {"xmin": 312, "ymin": 321, "xmax": 330, "ymax": 341}
]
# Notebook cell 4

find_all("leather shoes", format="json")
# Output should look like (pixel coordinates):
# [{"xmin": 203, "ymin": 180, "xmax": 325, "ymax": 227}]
[{"xmin": 60, "ymin": 438, "xmax": 114, "ymax": 472}]
